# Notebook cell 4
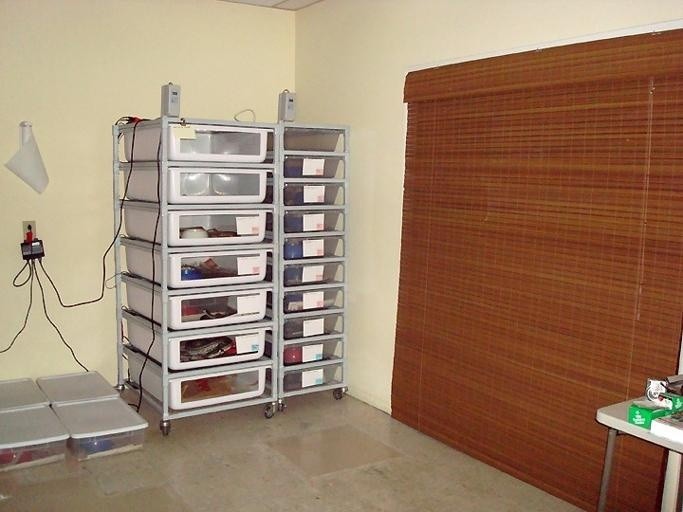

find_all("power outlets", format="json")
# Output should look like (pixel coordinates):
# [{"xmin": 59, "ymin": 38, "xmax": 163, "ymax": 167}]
[{"xmin": 22, "ymin": 220, "xmax": 36, "ymax": 242}]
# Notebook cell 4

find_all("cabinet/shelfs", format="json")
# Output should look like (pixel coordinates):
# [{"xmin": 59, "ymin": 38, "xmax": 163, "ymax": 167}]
[
  {"xmin": 114, "ymin": 115, "xmax": 279, "ymax": 436},
  {"xmin": 280, "ymin": 121, "xmax": 349, "ymax": 412}
]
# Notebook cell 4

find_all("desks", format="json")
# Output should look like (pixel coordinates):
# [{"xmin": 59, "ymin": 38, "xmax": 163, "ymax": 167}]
[{"xmin": 597, "ymin": 397, "xmax": 682, "ymax": 512}]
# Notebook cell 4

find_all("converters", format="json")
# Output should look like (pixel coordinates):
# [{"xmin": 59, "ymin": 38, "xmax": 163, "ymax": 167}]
[
  {"xmin": 32, "ymin": 240, "xmax": 44, "ymax": 258},
  {"xmin": 21, "ymin": 243, "xmax": 34, "ymax": 259}
]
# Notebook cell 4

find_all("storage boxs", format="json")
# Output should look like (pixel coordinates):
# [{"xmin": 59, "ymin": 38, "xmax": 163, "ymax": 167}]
[
  {"xmin": 1, "ymin": 377, "xmax": 50, "ymax": 410},
  {"xmin": 37, "ymin": 371, "xmax": 120, "ymax": 406},
  {"xmin": 1, "ymin": 408, "xmax": 70, "ymax": 471},
  {"xmin": 51, "ymin": 395, "xmax": 147, "ymax": 460}
]
